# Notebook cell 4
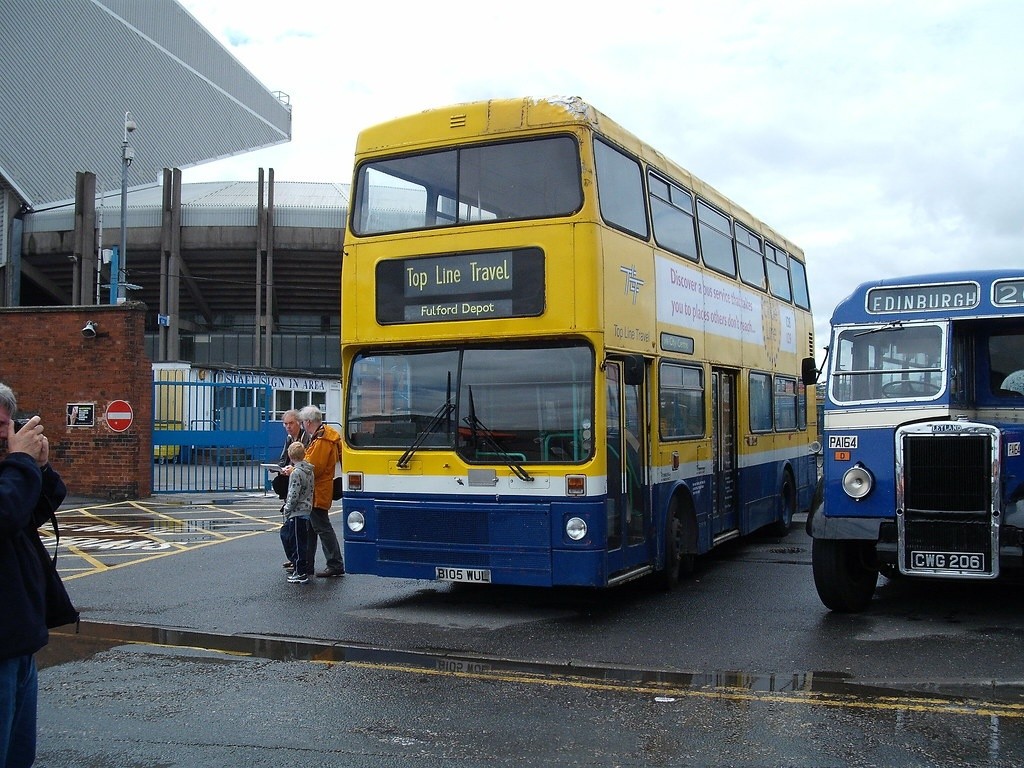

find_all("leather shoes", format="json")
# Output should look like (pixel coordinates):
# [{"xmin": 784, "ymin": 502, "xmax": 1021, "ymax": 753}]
[{"xmin": 283, "ymin": 561, "xmax": 294, "ymax": 567}]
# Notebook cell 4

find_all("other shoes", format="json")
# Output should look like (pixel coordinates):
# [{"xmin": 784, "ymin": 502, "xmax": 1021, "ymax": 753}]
[
  {"xmin": 316, "ymin": 568, "xmax": 345, "ymax": 578},
  {"xmin": 287, "ymin": 568, "xmax": 314, "ymax": 575}
]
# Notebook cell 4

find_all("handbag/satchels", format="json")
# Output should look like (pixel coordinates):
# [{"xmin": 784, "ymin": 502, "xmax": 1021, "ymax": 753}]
[
  {"xmin": 273, "ymin": 475, "xmax": 289, "ymax": 499},
  {"xmin": 332, "ymin": 477, "xmax": 344, "ymax": 500}
]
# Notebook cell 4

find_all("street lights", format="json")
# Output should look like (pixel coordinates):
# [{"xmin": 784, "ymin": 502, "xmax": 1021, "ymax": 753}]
[{"xmin": 116, "ymin": 111, "xmax": 137, "ymax": 300}]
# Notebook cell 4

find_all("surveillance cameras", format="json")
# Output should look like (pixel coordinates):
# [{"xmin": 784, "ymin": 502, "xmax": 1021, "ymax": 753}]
[{"xmin": 126, "ymin": 121, "xmax": 136, "ymax": 132}]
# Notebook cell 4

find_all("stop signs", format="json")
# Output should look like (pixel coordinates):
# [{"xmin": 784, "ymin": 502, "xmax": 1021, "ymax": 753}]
[{"xmin": 105, "ymin": 399, "xmax": 134, "ymax": 433}]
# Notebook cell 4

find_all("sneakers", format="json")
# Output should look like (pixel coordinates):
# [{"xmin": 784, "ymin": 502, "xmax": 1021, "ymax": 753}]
[{"xmin": 287, "ymin": 574, "xmax": 309, "ymax": 583}]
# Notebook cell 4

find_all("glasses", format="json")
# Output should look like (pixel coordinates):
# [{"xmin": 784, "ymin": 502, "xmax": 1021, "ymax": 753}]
[{"xmin": 283, "ymin": 420, "xmax": 299, "ymax": 427}]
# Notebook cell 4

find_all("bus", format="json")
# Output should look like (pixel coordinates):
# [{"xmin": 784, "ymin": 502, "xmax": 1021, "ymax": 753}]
[
  {"xmin": 331, "ymin": 93, "xmax": 822, "ymax": 590},
  {"xmin": 801, "ymin": 269, "xmax": 1024, "ymax": 612}
]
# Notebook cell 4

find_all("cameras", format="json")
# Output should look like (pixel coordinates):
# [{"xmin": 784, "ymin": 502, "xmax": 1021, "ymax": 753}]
[{"xmin": 13, "ymin": 418, "xmax": 31, "ymax": 434}]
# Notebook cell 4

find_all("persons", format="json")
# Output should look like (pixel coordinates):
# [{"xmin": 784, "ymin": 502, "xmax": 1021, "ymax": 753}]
[
  {"xmin": 0, "ymin": 382, "xmax": 78, "ymax": 768},
  {"xmin": 268, "ymin": 406, "xmax": 345, "ymax": 583}
]
